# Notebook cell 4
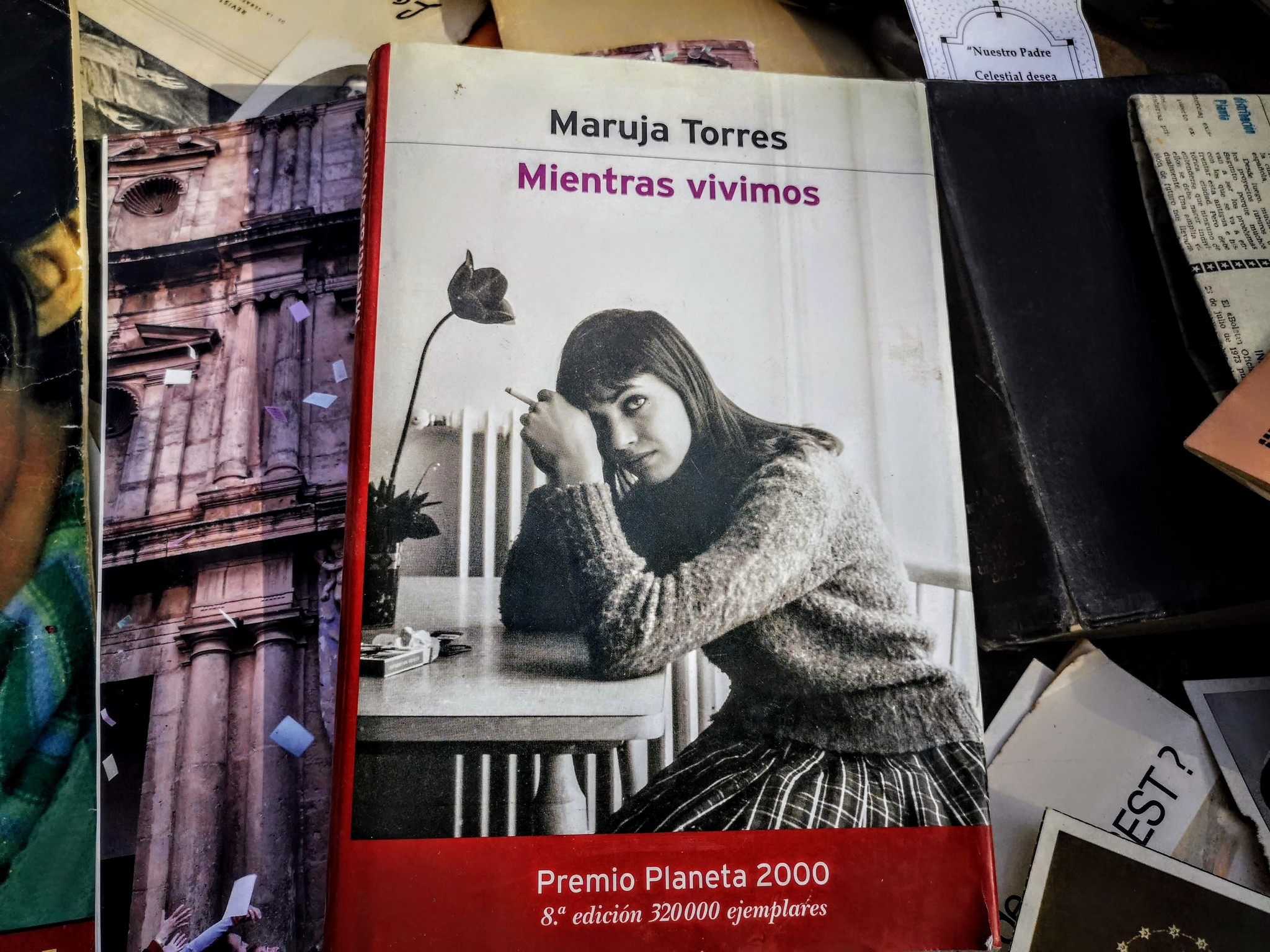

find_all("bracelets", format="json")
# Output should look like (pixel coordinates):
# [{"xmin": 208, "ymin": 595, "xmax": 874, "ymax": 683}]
[{"xmin": 315, "ymin": 944, "xmax": 321, "ymax": 951}]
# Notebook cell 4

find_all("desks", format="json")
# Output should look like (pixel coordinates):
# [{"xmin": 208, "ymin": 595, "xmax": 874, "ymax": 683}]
[{"xmin": 357, "ymin": 624, "xmax": 667, "ymax": 835}]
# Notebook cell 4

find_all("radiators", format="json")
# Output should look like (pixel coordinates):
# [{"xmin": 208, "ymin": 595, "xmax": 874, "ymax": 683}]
[{"xmin": 418, "ymin": 410, "xmax": 700, "ymax": 837}]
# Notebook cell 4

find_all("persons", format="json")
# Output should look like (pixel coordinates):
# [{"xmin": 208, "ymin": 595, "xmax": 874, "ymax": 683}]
[
  {"xmin": 497, "ymin": 309, "xmax": 988, "ymax": 834},
  {"xmin": 141, "ymin": 904, "xmax": 324, "ymax": 952}
]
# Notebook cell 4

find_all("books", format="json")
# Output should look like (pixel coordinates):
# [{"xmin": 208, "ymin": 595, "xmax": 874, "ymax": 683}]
[{"xmin": 327, "ymin": 45, "xmax": 1005, "ymax": 952}]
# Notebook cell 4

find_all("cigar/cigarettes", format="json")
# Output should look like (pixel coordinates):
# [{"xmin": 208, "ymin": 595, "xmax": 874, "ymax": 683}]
[{"xmin": 505, "ymin": 387, "xmax": 536, "ymax": 406}]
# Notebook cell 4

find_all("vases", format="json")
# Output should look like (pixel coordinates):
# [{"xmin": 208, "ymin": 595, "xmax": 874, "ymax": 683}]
[{"xmin": 363, "ymin": 479, "xmax": 440, "ymax": 629}]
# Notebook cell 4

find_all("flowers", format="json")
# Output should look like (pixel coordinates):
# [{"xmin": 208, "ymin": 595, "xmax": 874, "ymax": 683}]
[{"xmin": 368, "ymin": 249, "xmax": 516, "ymax": 546}]
